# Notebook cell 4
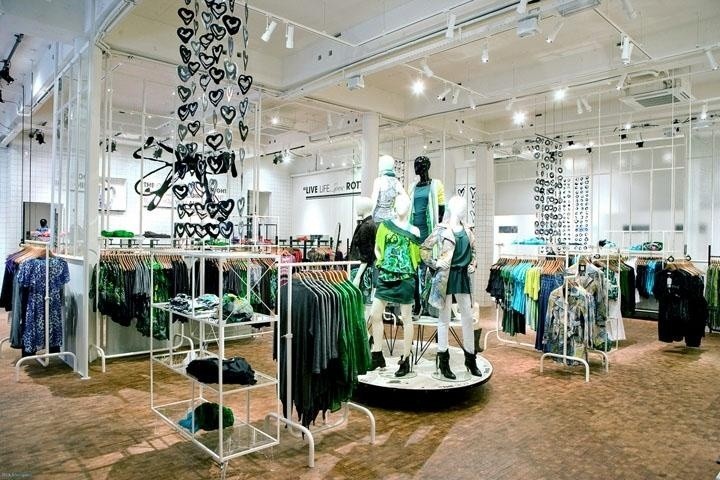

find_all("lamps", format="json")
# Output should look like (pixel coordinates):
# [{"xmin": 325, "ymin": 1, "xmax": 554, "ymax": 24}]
[
  {"xmin": 446, "ymin": 14, "xmax": 456, "ymax": 37},
  {"xmin": 621, "ymin": 36, "xmax": 634, "ymax": 66},
  {"xmin": 544, "ymin": 19, "xmax": 564, "ymax": 44},
  {"xmin": 260, "ymin": 21, "xmax": 295, "ymax": 49},
  {"xmin": 575, "ymin": 96, "xmax": 592, "ymax": 115},
  {"xmin": 437, "ymin": 88, "xmax": 476, "ymax": 110},
  {"xmin": 703, "ymin": 48, "xmax": 719, "ymax": 71}
]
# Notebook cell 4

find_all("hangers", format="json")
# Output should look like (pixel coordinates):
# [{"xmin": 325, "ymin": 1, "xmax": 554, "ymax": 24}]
[
  {"xmin": 99, "ymin": 248, "xmax": 278, "ymax": 272},
  {"xmin": 489, "ymin": 250, "xmax": 720, "ymax": 279},
  {"xmin": 283, "ymin": 261, "xmax": 351, "ymax": 284},
  {"xmin": 5, "ymin": 244, "xmax": 54, "ymax": 264}
]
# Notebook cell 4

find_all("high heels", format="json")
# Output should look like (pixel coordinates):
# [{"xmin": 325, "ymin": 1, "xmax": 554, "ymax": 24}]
[
  {"xmin": 437, "ymin": 350, "xmax": 457, "ymax": 380},
  {"xmin": 464, "ymin": 351, "xmax": 482, "ymax": 376}
]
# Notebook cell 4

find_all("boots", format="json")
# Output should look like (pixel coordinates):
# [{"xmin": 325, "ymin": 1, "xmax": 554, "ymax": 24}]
[
  {"xmin": 367, "ymin": 351, "xmax": 386, "ymax": 371},
  {"xmin": 395, "ymin": 354, "xmax": 410, "ymax": 376}
]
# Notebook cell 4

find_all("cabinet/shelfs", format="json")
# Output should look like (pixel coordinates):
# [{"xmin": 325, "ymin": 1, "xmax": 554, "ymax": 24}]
[{"xmin": 150, "ymin": 252, "xmax": 281, "ymax": 479}]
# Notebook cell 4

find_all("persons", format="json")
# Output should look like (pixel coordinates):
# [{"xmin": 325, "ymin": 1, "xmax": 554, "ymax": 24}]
[{"xmin": 348, "ymin": 153, "xmax": 483, "ymax": 379}]
